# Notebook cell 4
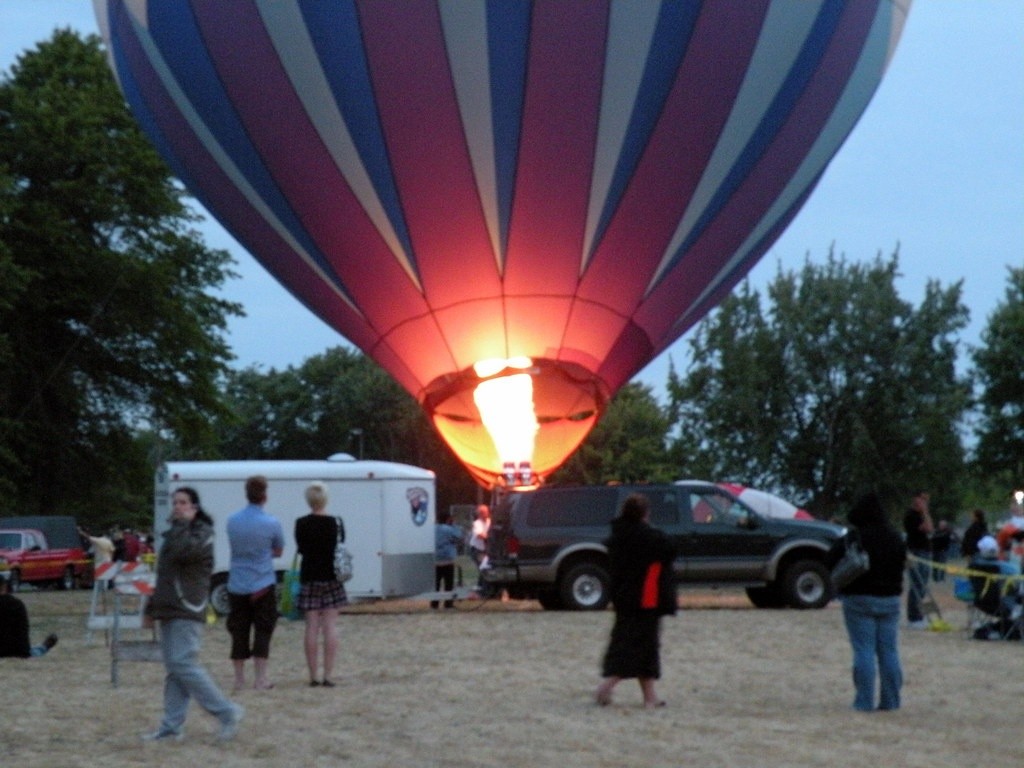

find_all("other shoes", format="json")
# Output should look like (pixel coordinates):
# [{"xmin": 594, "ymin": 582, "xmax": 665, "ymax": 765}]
[
  {"xmin": 323, "ymin": 679, "xmax": 335, "ymax": 686},
  {"xmin": 216, "ymin": 704, "xmax": 246, "ymax": 741},
  {"xmin": 43, "ymin": 635, "xmax": 59, "ymax": 649},
  {"xmin": 645, "ymin": 701, "xmax": 665, "ymax": 708},
  {"xmin": 596, "ymin": 694, "xmax": 610, "ymax": 704},
  {"xmin": 310, "ymin": 680, "xmax": 319, "ymax": 686},
  {"xmin": 256, "ymin": 681, "xmax": 277, "ymax": 690},
  {"xmin": 140, "ymin": 730, "xmax": 184, "ymax": 744},
  {"xmin": 908, "ymin": 621, "xmax": 929, "ymax": 630}
]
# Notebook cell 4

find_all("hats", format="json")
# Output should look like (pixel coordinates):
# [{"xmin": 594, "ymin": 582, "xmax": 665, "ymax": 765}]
[{"xmin": 977, "ymin": 535, "xmax": 999, "ymax": 558}]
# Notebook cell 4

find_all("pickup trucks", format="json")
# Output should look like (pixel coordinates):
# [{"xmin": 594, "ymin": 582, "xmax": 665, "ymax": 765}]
[{"xmin": 0, "ymin": 528, "xmax": 87, "ymax": 591}]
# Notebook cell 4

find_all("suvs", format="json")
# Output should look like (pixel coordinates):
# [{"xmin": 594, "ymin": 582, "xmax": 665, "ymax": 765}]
[{"xmin": 476, "ymin": 482, "xmax": 850, "ymax": 612}]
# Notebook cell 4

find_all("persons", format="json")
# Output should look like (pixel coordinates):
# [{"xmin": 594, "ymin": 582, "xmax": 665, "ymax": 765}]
[
  {"xmin": 0, "ymin": 579, "xmax": 58, "ymax": 659},
  {"xmin": 594, "ymin": 495, "xmax": 678, "ymax": 709},
  {"xmin": 139, "ymin": 487, "xmax": 242, "ymax": 746},
  {"xmin": 294, "ymin": 486, "xmax": 348, "ymax": 688},
  {"xmin": 77, "ymin": 524, "xmax": 155, "ymax": 594},
  {"xmin": 226, "ymin": 476, "xmax": 284, "ymax": 690},
  {"xmin": 468, "ymin": 504, "xmax": 493, "ymax": 596},
  {"xmin": 827, "ymin": 491, "xmax": 907, "ymax": 711},
  {"xmin": 901, "ymin": 488, "xmax": 1024, "ymax": 632},
  {"xmin": 429, "ymin": 509, "xmax": 463, "ymax": 607}
]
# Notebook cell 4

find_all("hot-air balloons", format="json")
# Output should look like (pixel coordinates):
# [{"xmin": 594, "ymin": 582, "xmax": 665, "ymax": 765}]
[{"xmin": 91, "ymin": 0, "xmax": 914, "ymax": 496}]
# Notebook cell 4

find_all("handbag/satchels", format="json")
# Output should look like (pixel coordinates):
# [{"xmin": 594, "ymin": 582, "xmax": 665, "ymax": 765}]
[
  {"xmin": 830, "ymin": 536, "xmax": 869, "ymax": 588},
  {"xmin": 334, "ymin": 516, "xmax": 353, "ymax": 582},
  {"xmin": 276, "ymin": 552, "xmax": 304, "ymax": 621},
  {"xmin": 642, "ymin": 563, "xmax": 680, "ymax": 614}
]
